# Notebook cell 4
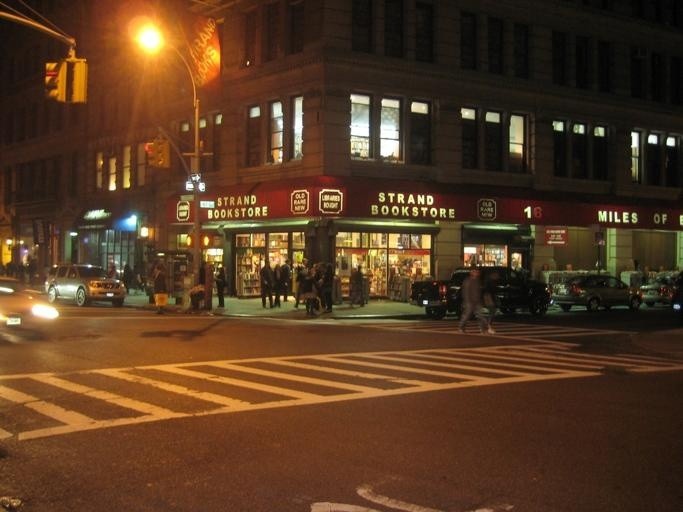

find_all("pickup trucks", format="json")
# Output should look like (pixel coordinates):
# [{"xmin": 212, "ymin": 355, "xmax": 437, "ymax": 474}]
[{"xmin": 410, "ymin": 266, "xmax": 551, "ymax": 319}]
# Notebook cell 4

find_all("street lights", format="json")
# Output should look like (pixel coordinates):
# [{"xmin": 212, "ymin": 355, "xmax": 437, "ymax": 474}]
[{"xmin": 136, "ymin": 22, "xmax": 201, "ymax": 312}]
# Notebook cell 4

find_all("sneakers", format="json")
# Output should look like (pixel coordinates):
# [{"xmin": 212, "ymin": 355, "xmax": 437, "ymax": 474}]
[
  {"xmin": 293, "ymin": 305, "xmax": 332, "ymax": 317},
  {"xmin": 458, "ymin": 325, "xmax": 495, "ymax": 336}
]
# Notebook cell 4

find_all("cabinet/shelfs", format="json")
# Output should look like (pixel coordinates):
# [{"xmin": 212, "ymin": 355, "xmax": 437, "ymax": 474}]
[
  {"xmin": 235, "ymin": 234, "xmax": 265, "ymax": 298},
  {"xmin": 340, "ymin": 273, "xmax": 408, "ymax": 301}
]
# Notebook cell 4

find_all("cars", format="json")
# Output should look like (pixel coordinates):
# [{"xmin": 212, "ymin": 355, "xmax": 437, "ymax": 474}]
[
  {"xmin": 45, "ymin": 263, "xmax": 126, "ymax": 306},
  {"xmin": 0, "ymin": 276, "xmax": 59, "ymax": 331},
  {"xmin": 551, "ymin": 274, "xmax": 641, "ymax": 311},
  {"xmin": 639, "ymin": 270, "xmax": 683, "ymax": 306}
]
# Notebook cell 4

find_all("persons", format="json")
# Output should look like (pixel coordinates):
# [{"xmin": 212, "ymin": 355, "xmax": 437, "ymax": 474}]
[
  {"xmin": 348, "ymin": 265, "xmax": 366, "ymax": 308},
  {"xmin": 257, "ymin": 258, "xmax": 334, "ymax": 317},
  {"xmin": 213, "ymin": 267, "xmax": 228, "ymax": 308},
  {"xmin": 457, "ymin": 265, "xmax": 500, "ymax": 335},
  {"xmin": 123, "ymin": 264, "xmax": 133, "ymax": 294},
  {"xmin": 109, "ymin": 263, "xmax": 117, "ymax": 277}
]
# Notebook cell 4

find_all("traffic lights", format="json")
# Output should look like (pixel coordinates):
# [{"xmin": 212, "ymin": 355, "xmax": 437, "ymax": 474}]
[{"xmin": 45, "ymin": 62, "xmax": 67, "ymax": 104}]
